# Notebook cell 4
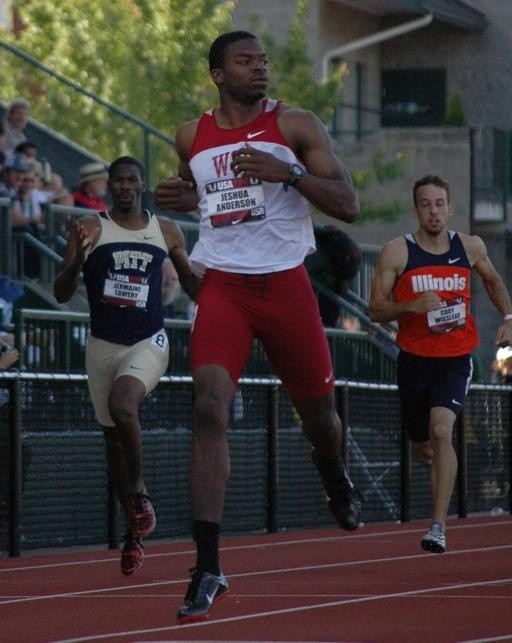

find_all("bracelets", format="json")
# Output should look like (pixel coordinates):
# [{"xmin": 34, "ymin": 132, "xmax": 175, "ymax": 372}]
[{"xmin": 503, "ymin": 313, "xmax": 512, "ymax": 321}]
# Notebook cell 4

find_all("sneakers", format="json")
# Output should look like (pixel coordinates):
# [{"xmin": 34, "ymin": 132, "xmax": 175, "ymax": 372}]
[
  {"xmin": 176, "ymin": 565, "xmax": 230, "ymax": 625},
  {"xmin": 420, "ymin": 521, "xmax": 446, "ymax": 554},
  {"xmin": 316, "ymin": 455, "xmax": 362, "ymax": 532},
  {"xmin": 120, "ymin": 529, "xmax": 144, "ymax": 577},
  {"xmin": 127, "ymin": 487, "xmax": 156, "ymax": 535}
]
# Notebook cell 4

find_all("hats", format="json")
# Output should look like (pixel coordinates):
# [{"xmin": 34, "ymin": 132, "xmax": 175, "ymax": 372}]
[{"xmin": 77, "ymin": 163, "xmax": 108, "ymax": 184}]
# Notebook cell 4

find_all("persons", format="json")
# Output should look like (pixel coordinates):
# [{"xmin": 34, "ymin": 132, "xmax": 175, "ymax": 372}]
[
  {"xmin": 1, "ymin": 93, "xmax": 361, "ymax": 373},
  {"xmin": 152, "ymin": 29, "xmax": 364, "ymax": 625},
  {"xmin": 367, "ymin": 172, "xmax": 512, "ymax": 553},
  {"xmin": 54, "ymin": 154, "xmax": 200, "ymax": 577}
]
